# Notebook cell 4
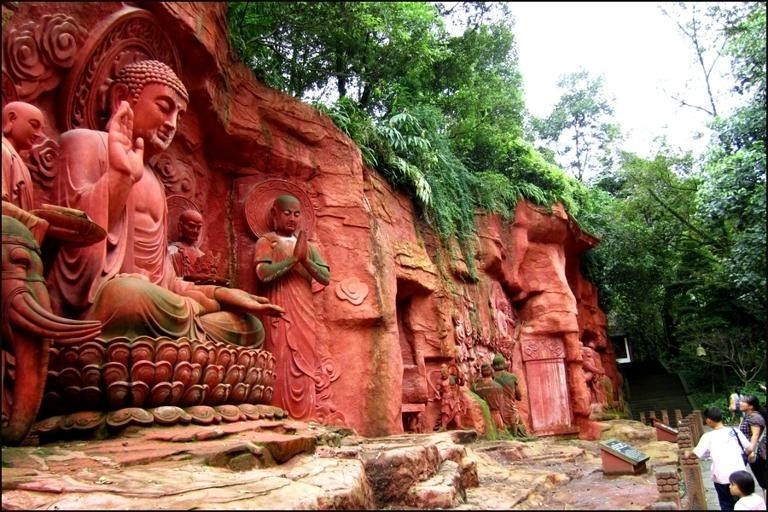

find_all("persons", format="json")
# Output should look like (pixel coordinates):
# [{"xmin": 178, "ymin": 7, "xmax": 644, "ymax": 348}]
[
  {"xmin": 729, "ymin": 385, "xmax": 745, "ymax": 422},
  {"xmin": 438, "ymin": 298, "xmax": 522, "ymax": 432},
  {"xmin": 2, "ymin": 102, "xmax": 80, "ymax": 246},
  {"xmin": 739, "ymin": 393, "xmax": 766, "ymax": 490},
  {"xmin": 171, "ymin": 210, "xmax": 205, "ymax": 279},
  {"xmin": 729, "ymin": 472, "xmax": 766, "ymax": 510},
  {"xmin": 55, "ymin": 61, "xmax": 290, "ymax": 343},
  {"xmin": 684, "ymin": 406, "xmax": 755, "ymax": 510},
  {"xmin": 253, "ymin": 195, "xmax": 331, "ymax": 419},
  {"xmin": 581, "ymin": 332, "xmax": 612, "ymax": 410}
]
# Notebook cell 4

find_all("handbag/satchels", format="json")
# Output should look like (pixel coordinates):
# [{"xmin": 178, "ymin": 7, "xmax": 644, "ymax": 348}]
[{"xmin": 740, "ymin": 450, "xmax": 750, "ymax": 467}]
